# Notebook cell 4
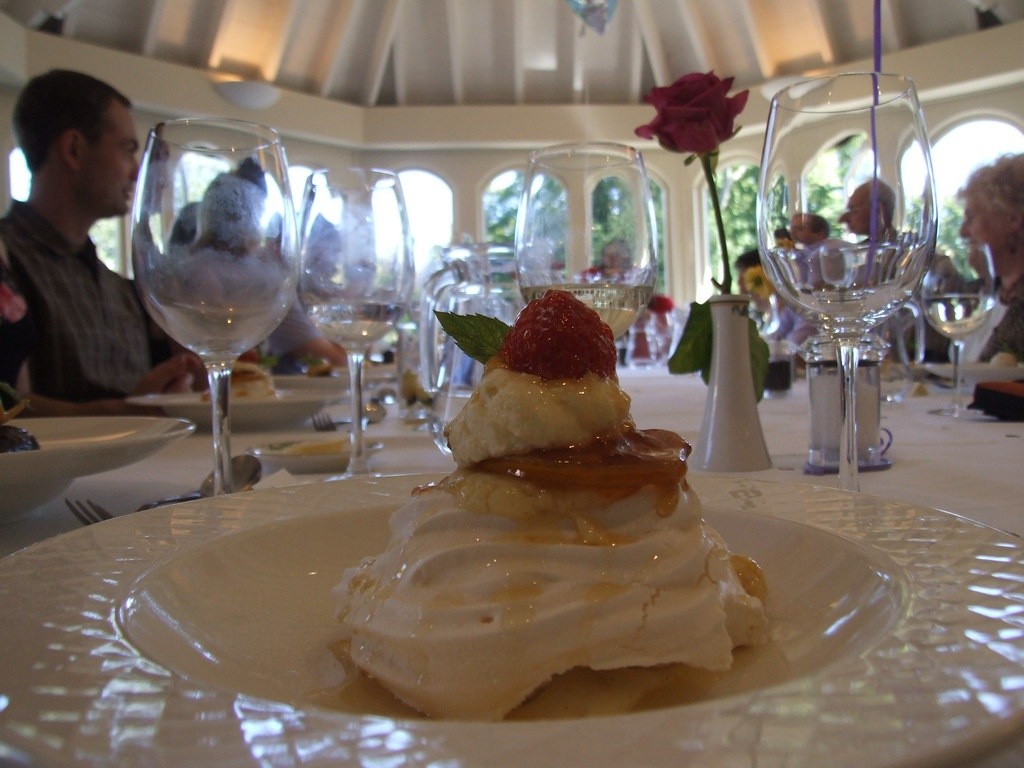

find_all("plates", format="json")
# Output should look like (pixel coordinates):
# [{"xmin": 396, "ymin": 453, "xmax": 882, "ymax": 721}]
[
  {"xmin": 0, "ymin": 472, "xmax": 1024, "ymax": 768},
  {"xmin": 925, "ymin": 362, "xmax": 1024, "ymax": 383}
]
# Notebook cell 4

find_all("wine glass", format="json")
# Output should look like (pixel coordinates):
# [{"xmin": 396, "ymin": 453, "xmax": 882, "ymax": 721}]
[
  {"xmin": 128, "ymin": 116, "xmax": 302, "ymax": 499},
  {"xmin": 754, "ymin": 73, "xmax": 939, "ymax": 494},
  {"xmin": 293, "ymin": 166, "xmax": 415, "ymax": 483},
  {"xmin": 775, "ymin": 242, "xmax": 998, "ymax": 422}
]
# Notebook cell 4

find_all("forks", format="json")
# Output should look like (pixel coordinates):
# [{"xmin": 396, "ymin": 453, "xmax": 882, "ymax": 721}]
[
  {"xmin": 64, "ymin": 499, "xmax": 114, "ymax": 526},
  {"xmin": 310, "ymin": 411, "xmax": 337, "ymax": 432}
]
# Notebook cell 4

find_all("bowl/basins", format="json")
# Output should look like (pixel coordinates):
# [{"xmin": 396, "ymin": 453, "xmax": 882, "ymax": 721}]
[
  {"xmin": 127, "ymin": 374, "xmax": 350, "ymax": 432},
  {"xmin": 0, "ymin": 416, "xmax": 197, "ymax": 524}
]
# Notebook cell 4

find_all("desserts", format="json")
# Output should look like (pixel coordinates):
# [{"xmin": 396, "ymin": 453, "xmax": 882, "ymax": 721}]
[
  {"xmin": 329, "ymin": 285, "xmax": 768, "ymax": 721},
  {"xmin": 202, "ymin": 347, "xmax": 278, "ymax": 402}
]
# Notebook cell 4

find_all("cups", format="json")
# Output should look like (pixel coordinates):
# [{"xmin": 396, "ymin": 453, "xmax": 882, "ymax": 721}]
[{"xmin": 511, "ymin": 138, "xmax": 658, "ymax": 342}]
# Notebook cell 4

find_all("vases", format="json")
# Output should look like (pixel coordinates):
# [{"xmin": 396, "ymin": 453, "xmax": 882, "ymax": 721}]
[{"xmin": 690, "ymin": 293, "xmax": 773, "ymax": 475}]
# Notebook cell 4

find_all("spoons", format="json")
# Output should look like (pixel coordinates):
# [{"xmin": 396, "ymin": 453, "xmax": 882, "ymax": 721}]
[
  {"xmin": 136, "ymin": 454, "xmax": 263, "ymax": 513},
  {"xmin": 360, "ymin": 401, "xmax": 386, "ymax": 430}
]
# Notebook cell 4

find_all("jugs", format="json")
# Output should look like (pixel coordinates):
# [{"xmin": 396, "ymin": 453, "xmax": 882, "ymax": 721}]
[{"xmin": 414, "ymin": 239, "xmax": 519, "ymax": 459}]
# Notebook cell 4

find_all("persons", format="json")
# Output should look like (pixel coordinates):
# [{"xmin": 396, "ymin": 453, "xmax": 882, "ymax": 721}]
[
  {"xmin": 601, "ymin": 238, "xmax": 633, "ymax": 275},
  {"xmin": 956, "ymin": 152, "xmax": 1024, "ymax": 382},
  {"xmin": 735, "ymin": 245, "xmax": 816, "ymax": 372},
  {"xmin": 0, "ymin": 69, "xmax": 260, "ymax": 403},
  {"xmin": 774, "ymin": 179, "xmax": 972, "ymax": 365},
  {"xmin": 165, "ymin": 155, "xmax": 349, "ymax": 373}
]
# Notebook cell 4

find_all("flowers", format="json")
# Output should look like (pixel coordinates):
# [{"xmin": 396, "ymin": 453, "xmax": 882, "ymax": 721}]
[{"xmin": 635, "ymin": 70, "xmax": 770, "ymax": 406}]
